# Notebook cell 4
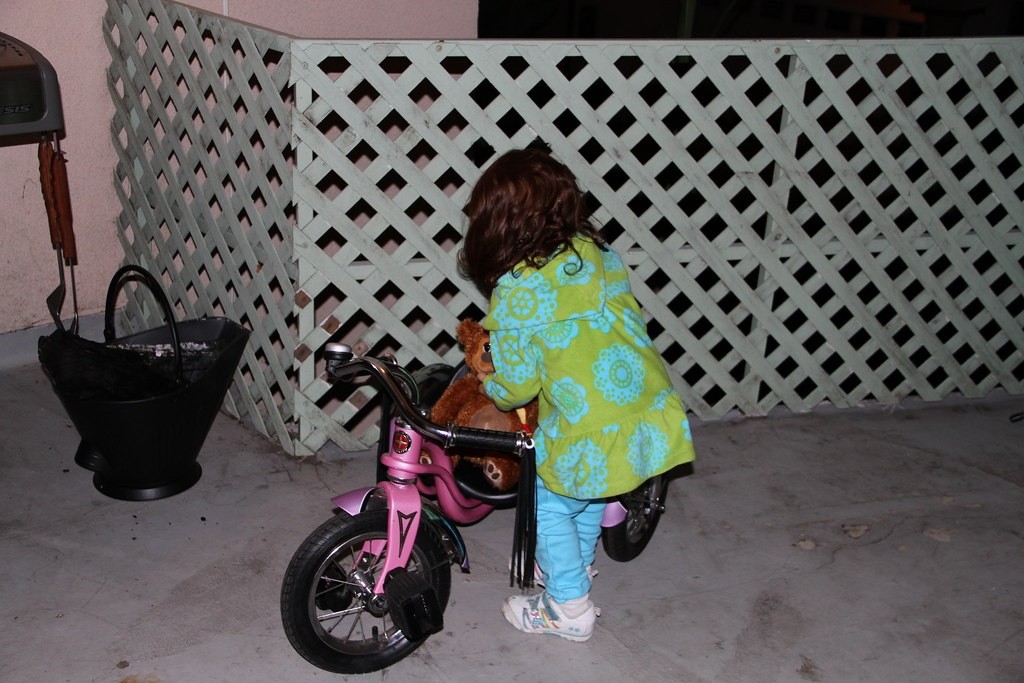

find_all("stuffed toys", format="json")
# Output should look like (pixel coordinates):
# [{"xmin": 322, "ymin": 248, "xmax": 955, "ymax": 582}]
[{"xmin": 419, "ymin": 320, "xmax": 538, "ymax": 491}]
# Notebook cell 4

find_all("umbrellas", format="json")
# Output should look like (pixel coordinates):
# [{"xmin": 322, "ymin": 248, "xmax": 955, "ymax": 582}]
[{"xmin": 38, "ymin": 132, "xmax": 79, "ymax": 336}]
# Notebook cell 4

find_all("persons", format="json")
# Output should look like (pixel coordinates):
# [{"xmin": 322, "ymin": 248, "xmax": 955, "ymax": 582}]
[{"xmin": 458, "ymin": 149, "xmax": 697, "ymax": 643}]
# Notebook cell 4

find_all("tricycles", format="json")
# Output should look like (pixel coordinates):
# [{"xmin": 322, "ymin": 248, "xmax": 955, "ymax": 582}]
[{"xmin": 279, "ymin": 341, "xmax": 669, "ymax": 674}]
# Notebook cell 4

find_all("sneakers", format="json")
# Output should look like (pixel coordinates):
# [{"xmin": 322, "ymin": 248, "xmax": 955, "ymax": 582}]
[
  {"xmin": 502, "ymin": 591, "xmax": 601, "ymax": 642},
  {"xmin": 508, "ymin": 550, "xmax": 598, "ymax": 588}
]
